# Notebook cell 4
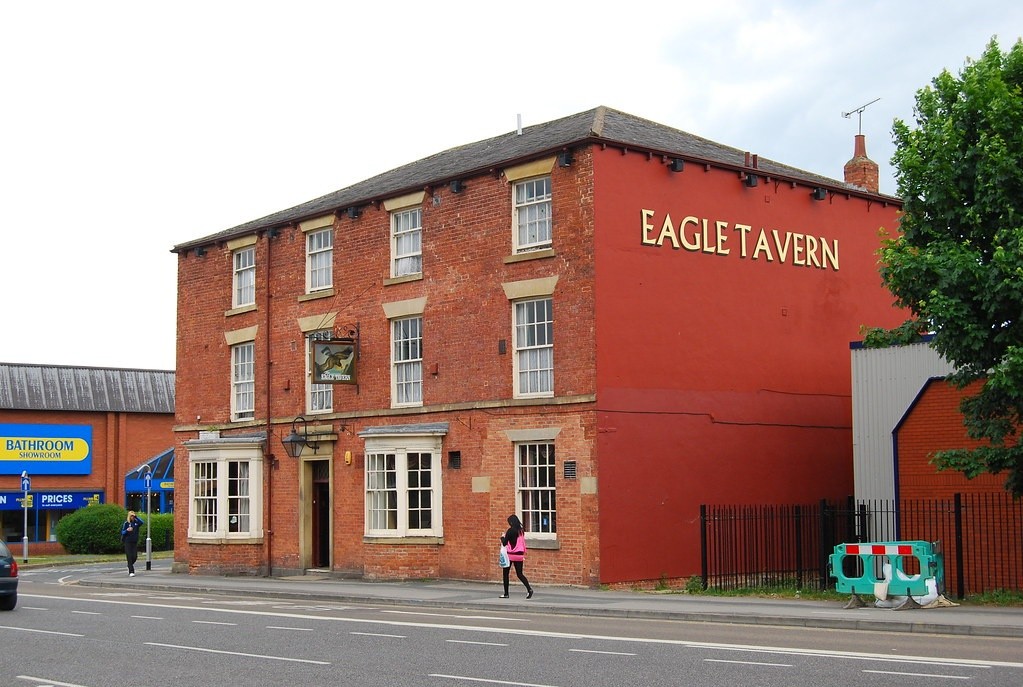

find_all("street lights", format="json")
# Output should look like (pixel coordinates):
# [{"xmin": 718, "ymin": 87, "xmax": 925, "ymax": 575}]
[
  {"xmin": 136, "ymin": 464, "xmax": 152, "ymax": 570},
  {"xmin": 20, "ymin": 470, "xmax": 28, "ymax": 563}
]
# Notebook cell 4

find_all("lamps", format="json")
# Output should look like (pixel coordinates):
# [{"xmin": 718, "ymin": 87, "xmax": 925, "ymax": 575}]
[
  {"xmin": 348, "ymin": 207, "xmax": 362, "ymax": 218},
  {"xmin": 267, "ymin": 229, "xmax": 281, "ymax": 239},
  {"xmin": 667, "ymin": 159, "xmax": 684, "ymax": 172},
  {"xmin": 559, "ymin": 153, "xmax": 577, "ymax": 167},
  {"xmin": 450, "ymin": 181, "xmax": 466, "ymax": 194},
  {"xmin": 281, "ymin": 416, "xmax": 319, "ymax": 458},
  {"xmin": 194, "ymin": 247, "xmax": 207, "ymax": 257},
  {"xmin": 743, "ymin": 175, "xmax": 758, "ymax": 187},
  {"xmin": 810, "ymin": 187, "xmax": 825, "ymax": 200}
]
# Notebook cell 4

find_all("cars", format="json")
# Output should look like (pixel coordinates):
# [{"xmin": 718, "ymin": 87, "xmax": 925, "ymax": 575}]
[{"xmin": 0, "ymin": 540, "xmax": 19, "ymax": 612}]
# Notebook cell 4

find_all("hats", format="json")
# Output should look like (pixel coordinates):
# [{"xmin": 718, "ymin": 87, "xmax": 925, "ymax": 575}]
[{"xmin": 127, "ymin": 511, "xmax": 136, "ymax": 523}]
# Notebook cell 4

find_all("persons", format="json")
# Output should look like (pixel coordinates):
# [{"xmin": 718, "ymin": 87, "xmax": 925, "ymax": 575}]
[
  {"xmin": 122, "ymin": 510, "xmax": 144, "ymax": 577},
  {"xmin": 499, "ymin": 515, "xmax": 534, "ymax": 599}
]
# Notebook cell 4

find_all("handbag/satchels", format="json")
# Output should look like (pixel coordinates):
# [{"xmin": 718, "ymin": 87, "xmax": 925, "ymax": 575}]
[
  {"xmin": 121, "ymin": 521, "xmax": 132, "ymax": 542},
  {"xmin": 499, "ymin": 540, "xmax": 510, "ymax": 568}
]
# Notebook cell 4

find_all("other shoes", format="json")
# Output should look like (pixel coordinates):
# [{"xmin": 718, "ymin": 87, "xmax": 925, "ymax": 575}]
[
  {"xmin": 499, "ymin": 594, "xmax": 509, "ymax": 598},
  {"xmin": 129, "ymin": 573, "xmax": 135, "ymax": 577},
  {"xmin": 526, "ymin": 589, "xmax": 533, "ymax": 598}
]
